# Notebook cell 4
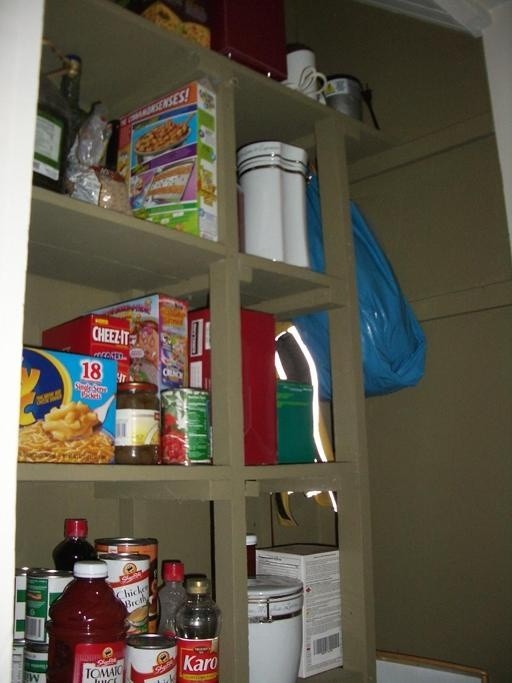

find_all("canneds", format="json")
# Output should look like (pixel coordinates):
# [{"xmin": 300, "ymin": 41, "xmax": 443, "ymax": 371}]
[
  {"xmin": 161, "ymin": 387, "xmax": 211, "ymax": 466},
  {"xmin": 11, "ymin": 568, "xmax": 74, "ymax": 682},
  {"xmin": 124, "ymin": 633, "xmax": 178, "ymax": 683},
  {"xmin": 93, "ymin": 536, "xmax": 159, "ymax": 632},
  {"xmin": 101, "ymin": 553, "xmax": 150, "ymax": 636}
]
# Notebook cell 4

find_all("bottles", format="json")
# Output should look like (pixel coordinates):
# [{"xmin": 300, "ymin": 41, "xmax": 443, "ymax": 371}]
[
  {"xmin": 46, "ymin": 517, "xmax": 221, "ymax": 683},
  {"xmin": 56, "ymin": 53, "xmax": 88, "ymax": 194}
]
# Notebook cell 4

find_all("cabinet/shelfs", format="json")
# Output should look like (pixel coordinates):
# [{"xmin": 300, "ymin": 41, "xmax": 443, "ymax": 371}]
[{"xmin": 2, "ymin": 0, "xmax": 394, "ymax": 682}]
